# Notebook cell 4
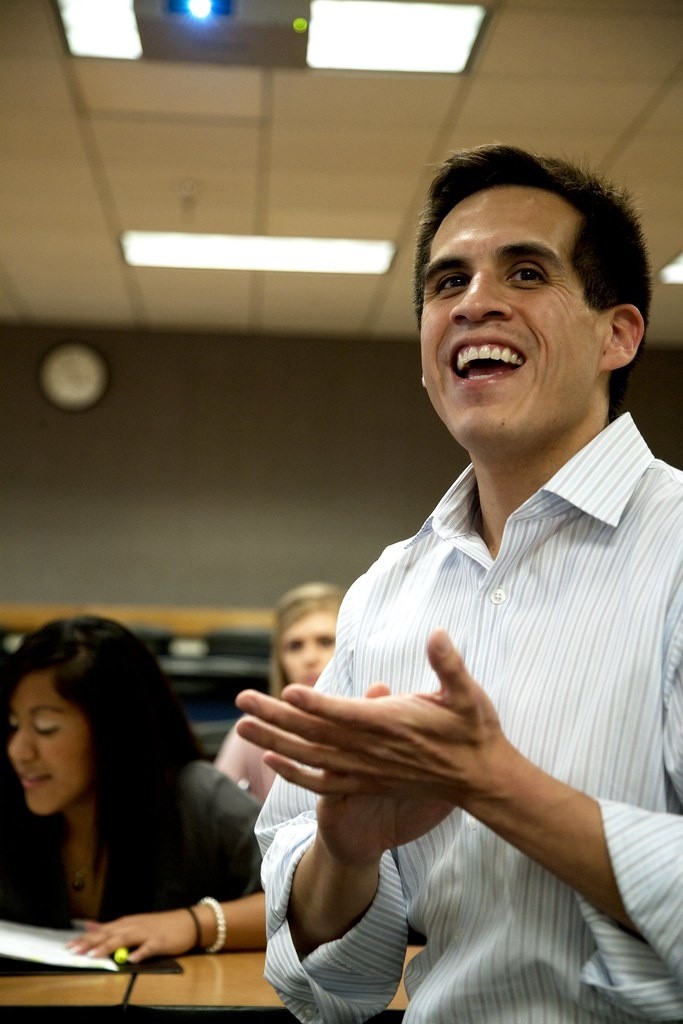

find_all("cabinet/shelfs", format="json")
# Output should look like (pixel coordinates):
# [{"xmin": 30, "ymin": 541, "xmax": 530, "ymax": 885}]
[{"xmin": 0, "ymin": 602, "xmax": 285, "ymax": 739}]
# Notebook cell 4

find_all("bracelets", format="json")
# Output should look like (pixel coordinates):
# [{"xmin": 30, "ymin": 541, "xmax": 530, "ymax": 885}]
[
  {"xmin": 199, "ymin": 896, "xmax": 226, "ymax": 953},
  {"xmin": 184, "ymin": 906, "xmax": 203, "ymax": 948}
]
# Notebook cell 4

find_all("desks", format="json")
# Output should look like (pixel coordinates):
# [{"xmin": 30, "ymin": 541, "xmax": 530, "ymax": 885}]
[{"xmin": 0, "ymin": 943, "xmax": 430, "ymax": 1024}]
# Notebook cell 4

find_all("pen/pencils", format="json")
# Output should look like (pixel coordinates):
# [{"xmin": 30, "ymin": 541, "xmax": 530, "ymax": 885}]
[{"xmin": 111, "ymin": 946, "xmax": 127, "ymax": 966}]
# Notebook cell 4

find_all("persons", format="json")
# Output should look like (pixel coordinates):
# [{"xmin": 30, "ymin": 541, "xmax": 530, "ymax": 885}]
[
  {"xmin": 0, "ymin": 617, "xmax": 266, "ymax": 960},
  {"xmin": 217, "ymin": 584, "xmax": 341, "ymax": 803},
  {"xmin": 233, "ymin": 146, "xmax": 682, "ymax": 1024}
]
